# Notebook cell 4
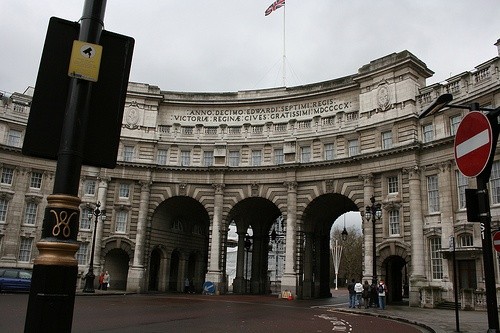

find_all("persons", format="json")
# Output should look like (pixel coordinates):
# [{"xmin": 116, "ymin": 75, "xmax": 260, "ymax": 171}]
[
  {"xmin": 354, "ymin": 278, "xmax": 364, "ymax": 309},
  {"xmin": 102, "ymin": 270, "xmax": 111, "ymax": 291},
  {"xmin": 369, "ymin": 279, "xmax": 380, "ymax": 308},
  {"xmin": 362, "ymin": 280, "xmax": 371, "ymax": 309},
  {"xmin": 184, "ymin": 277, "xmax": 189, "ymax": 294},
  {"xmin": 98, "ymin": 271, "xmax": 104, "ymax": 290},
  {"xmin": 348, "ymin": 278, "xmax": 356, "ymax": 308},
  {"xmin": 376, "ymin": 279, "xmax": 388, "ymax": 310}
]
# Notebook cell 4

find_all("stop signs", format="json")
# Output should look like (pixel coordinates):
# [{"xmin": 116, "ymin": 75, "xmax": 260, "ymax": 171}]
[
  {"xmin": 492, "ymin": 231, "xmax": 500, "ymax": 253},
  {"xmin": 454, "ymin": 111, "xmax": 493, "ymax": 178}
]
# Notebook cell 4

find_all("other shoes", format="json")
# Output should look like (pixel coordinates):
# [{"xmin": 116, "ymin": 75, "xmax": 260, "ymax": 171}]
[
  {"xmin": 348, "ymin": 306, "xmax": 351, "ymax": 308},
  {"xmin": 352, "ymin": 306, "xmax": 356, "ymax": 308},
  {"xmin": 365, "ymin": 307, "xmax": 367, "ymax": 309}
]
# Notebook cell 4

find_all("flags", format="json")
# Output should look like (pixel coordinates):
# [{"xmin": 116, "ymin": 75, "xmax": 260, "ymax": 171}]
[{"xmin": 264, "ymin": 0, "xmax": 285, "ymax": 16}]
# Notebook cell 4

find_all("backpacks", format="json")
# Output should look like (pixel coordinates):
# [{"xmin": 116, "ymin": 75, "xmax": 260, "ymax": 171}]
[
  {"xmin": 378, "ymin": 282, "xmax": 385, "ymax": 293},
  {"xmin": 367, "ymin": 285, "xmax": 372, "ymax": 292}
]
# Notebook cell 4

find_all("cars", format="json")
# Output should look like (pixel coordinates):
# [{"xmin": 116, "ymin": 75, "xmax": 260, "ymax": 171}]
[{"xmin": 0, "ymin": 267, "xmax": 33, "ymax": 295}]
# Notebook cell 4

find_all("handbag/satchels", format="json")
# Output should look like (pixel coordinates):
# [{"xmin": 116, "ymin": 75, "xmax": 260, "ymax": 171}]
[
  {"xmin": 97, "ymin": 284, "xmax": 100, "ymax": 289},
  {"xmin": 107, "ymin": 283, "xmax": 110, "ymax": 288}
]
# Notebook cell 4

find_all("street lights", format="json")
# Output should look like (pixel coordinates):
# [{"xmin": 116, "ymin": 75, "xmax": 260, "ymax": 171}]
[
  {"xmin": 82, "ymin": 201, "xmax": 106, "ymax": 293},
  {"xmin": 364, "ymin": 196, "xmax": 383, "ymax": 308}
]
